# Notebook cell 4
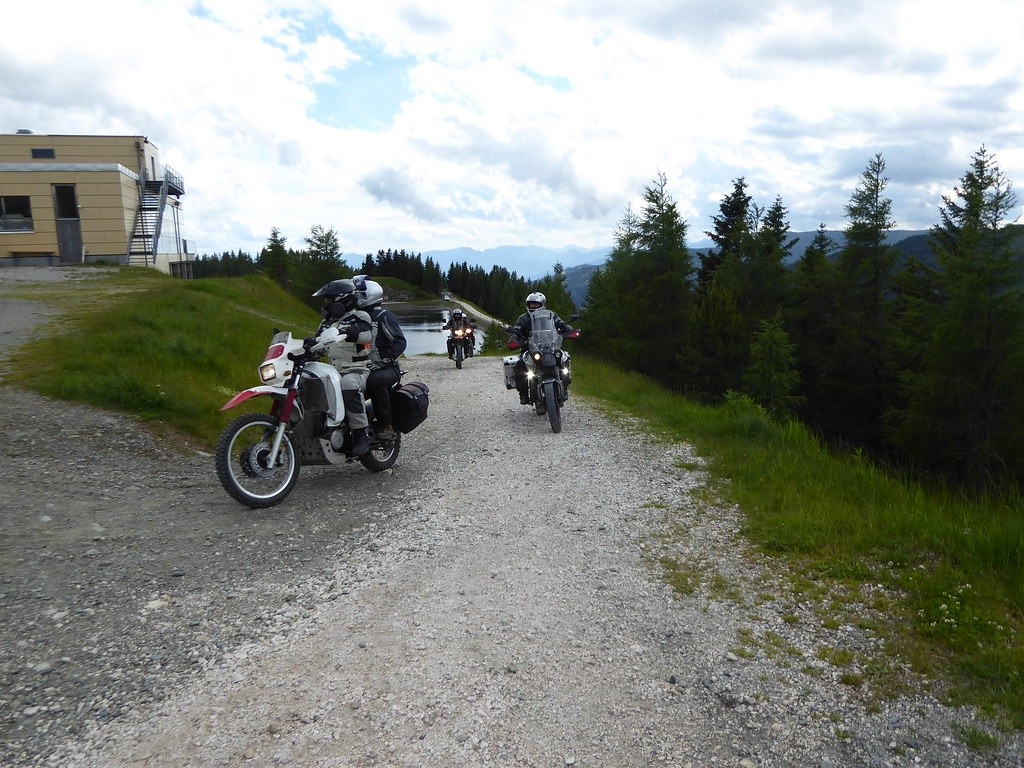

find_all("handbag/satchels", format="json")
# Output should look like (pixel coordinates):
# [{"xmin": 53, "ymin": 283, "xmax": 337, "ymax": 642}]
[{"xmin": 394, "ymin": 381, "xmax": 430, "ymax": 434}]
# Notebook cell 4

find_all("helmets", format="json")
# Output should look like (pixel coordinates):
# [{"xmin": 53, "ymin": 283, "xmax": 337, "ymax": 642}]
[
  {"xmin": 525, "ymin": 291, "xmax": 546, "ymax": 315},
  {"xmin": 462, "ymin": 313, "xmax": 467, "ymax": 319},
  {"xmin": 452, "ymin": 309, "xmax": 462, "ymax": 318},
  {"xmin": 353, "ymin": 280, "xmax": 384, "ymax": 309},
  {"xmin": 322, "ymin": 279, "xmax": 357, "ymax": 325}
]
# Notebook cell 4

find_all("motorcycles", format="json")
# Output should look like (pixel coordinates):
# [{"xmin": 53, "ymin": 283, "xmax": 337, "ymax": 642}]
[
  {"xmin": 214, "ymin": 325, "xmax": 409, "ymax": 508},
  {"xmin": 505, "ymin": 310, "xmax": 582, "ymax": 432},
  {"xmin": 441, "ymin": 318, "xmax": 477, "ymax": 369}
]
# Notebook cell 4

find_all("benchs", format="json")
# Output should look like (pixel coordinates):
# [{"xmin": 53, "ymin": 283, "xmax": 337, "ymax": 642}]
[{"xmin": 10, "ymin": 250, "xmax": 55, "ymax": 265}]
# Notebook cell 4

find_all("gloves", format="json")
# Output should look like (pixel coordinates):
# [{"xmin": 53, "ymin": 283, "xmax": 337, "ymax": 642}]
[
  {"xmin": 344, "ymin": 327, "xmax": 359, "ymax": 342},
  {"xmin": 570, "ymin": 331, "xmax": 582, "ymax": 340},
  {"xmin": 508, "ymin": 342, "xmax": 519, "ymax": 351},
  {"xmin": 366, "ymin": 358, "xmax": 392, "ymax": 371}
]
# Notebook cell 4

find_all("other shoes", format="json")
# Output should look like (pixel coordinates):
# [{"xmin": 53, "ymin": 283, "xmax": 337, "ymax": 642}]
[
  {"xmin": 465, "ymin": 354, "xmax": 469, "ymax": 358},
  {"xmin": 378, "ymin": 424, "xmax": 394, "ymax": 440},
  {"xmin": 449, "ymin": 353, "xmax": 454, "ymax": 359},
  {"xmin": 352, "ymin": 429, "xmax": 370, "ymax": 456},
  {"xmin": 521, "ymin": 394, "xmax": 530, "ymax": 404}
]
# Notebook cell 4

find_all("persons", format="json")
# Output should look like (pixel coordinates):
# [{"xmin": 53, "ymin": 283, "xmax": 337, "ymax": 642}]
[
  {"xmin": 508, "ymin": 292, "xmax": 580, "ymax": 405},
  {"xmin": 442, "ymin": 309, "xmax": 476, "ymax": 360},
  {"xmin": 304, "ymin": 278, "xmax": 377, "ymax": 456},
  {"xmin": 351, "ymin": 274, "xmax": 407, "ymax": 440}
]
